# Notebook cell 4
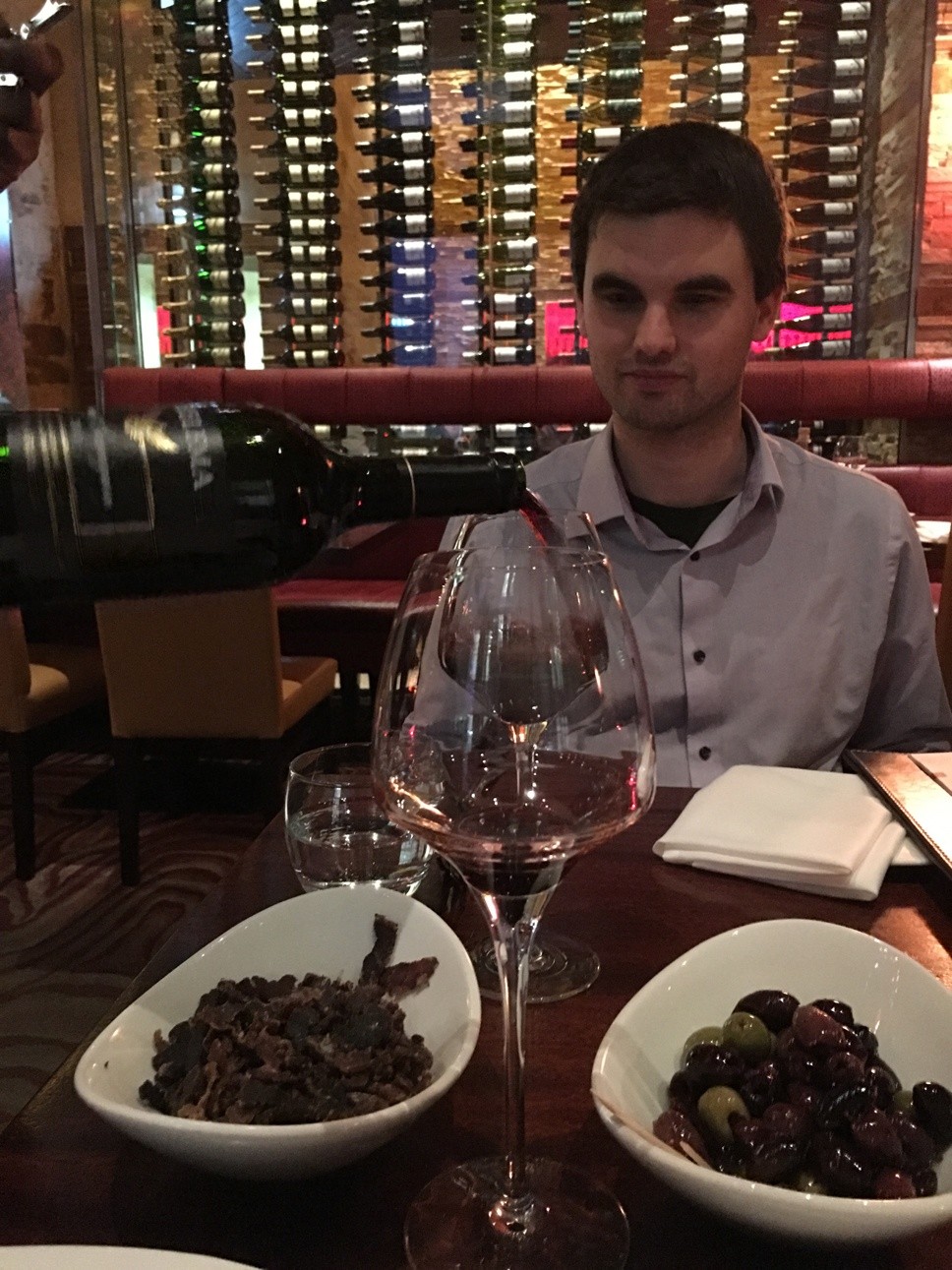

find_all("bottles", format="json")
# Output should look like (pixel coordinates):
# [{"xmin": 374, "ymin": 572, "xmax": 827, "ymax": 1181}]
[
  {"xmin": 315, "ymin": 422, "xmax": 858, "ymax": 460},
  {"xmin": 0, "ymin": 402, "xmax": 527, "ymax": 603},
  {"xmin": 150, "ymin": 0, "xmax": 874, "ymax": 371}
]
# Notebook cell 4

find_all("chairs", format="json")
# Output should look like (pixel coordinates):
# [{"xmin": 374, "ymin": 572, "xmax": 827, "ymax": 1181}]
[
  {"xmin": 0, "ymin": 593, "xmax": 111, "ymax": 880},
  {"xmin": 79, "ymin": 548, "xmax": 338, "ymax": 889}
]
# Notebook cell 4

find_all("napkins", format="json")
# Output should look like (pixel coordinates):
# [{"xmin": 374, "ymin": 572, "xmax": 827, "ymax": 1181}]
[{"xmin": 651, "ymin": 764, "xmax": 909, "ymax": 902}]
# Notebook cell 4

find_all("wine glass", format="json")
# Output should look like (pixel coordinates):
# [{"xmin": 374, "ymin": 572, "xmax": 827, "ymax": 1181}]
[{"xmin": 370, "ymin": 508, "xmax": 660, "ymax": 1270}]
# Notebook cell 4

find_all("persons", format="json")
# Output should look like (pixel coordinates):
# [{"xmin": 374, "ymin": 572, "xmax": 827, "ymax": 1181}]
[
  {"xmin": 0, "ymin": 12, "xmax": 65, "ymax": 192},
  {"xmin": 399, "ymin": 121, "xmax": 952, "ymax": 789}
]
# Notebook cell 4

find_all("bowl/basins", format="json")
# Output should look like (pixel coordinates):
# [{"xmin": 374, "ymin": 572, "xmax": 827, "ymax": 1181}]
[
  {"xmin": 590, "ymin": 918, "xmax": 951, "ymax": 1244},
  {"xmin": 74, "ymin": 886, "xmax": 481, "ymax": 1177}
]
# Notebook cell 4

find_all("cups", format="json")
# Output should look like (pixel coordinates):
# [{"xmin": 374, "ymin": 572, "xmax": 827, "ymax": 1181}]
[
  {"xmin": 283, "ymin": 744, "xmax": 431, "ymax": 896},
  {"xmin": 834, "ymin": 435, "xmax": 867, "ymax": 472}
]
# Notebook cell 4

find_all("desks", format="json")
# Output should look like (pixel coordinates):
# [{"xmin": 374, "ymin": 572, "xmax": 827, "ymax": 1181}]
[
  {"xmin": 271, "ymin": 577, "xmax": 443, "ymax": 742},
  {"xmin": 0, "ymin": 749, "xmax": 952, "ymax": 1270}
]
